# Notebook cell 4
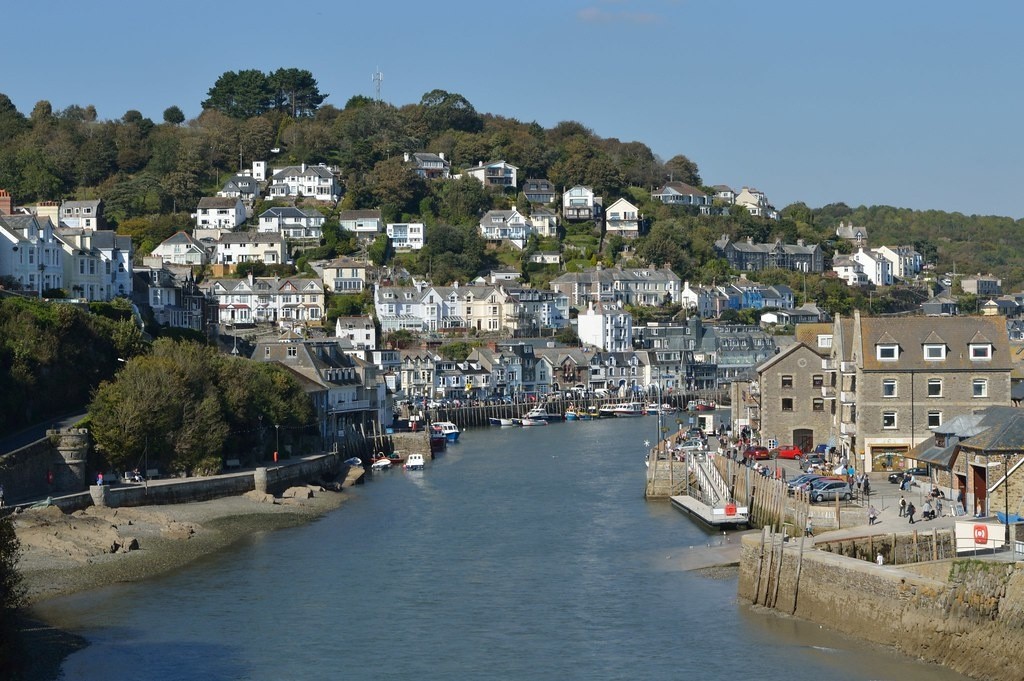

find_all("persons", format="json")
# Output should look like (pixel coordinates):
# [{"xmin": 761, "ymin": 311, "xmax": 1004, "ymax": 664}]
[
  {"xmin": 96, "ymin": 473, "xmax": 105, "ymax": 485},
  {"xmin": 807, "ymin": 461, "xmax": 982, "ymax": 566},
  {"xmin": 132, "ymin": 468, "xmax": 145, "ymax": 482},
  {"xmin": 667, "ymin": 419, "xmax": 773, "ymax": 478},
  {"xmin": 0, "ymin": 485, "xmax": 5, "ymax": 508}
]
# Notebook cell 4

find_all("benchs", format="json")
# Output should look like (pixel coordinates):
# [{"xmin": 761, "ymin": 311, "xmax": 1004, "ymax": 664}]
[
  {"xmin": 226, "ymin": 459, "xmax": 242, "ymax": 472},
  {"xmin": 125, "ymin": 472, "xmax": 138, "ymax": 483},
  {"xmin": 96, "ymin": 474, "xmax": 120, "ymax": 485},
  {"xmin": 146, "ymin": 469, "xmax": 161, "ymax": 480}
]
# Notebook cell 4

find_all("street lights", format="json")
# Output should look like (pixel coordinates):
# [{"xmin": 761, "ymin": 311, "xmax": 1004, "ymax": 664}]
[{"xmin": 275, "ymin": 423, "xmax": 280, "ymax": 453}]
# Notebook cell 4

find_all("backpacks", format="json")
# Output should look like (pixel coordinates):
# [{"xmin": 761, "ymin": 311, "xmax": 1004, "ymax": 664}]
[{"xmin": 901, "ymin": 498, "xmax": 906, "ymax": 505}]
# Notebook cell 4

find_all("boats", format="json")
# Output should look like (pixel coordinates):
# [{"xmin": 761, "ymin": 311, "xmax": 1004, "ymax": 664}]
[
  {"xmin": 697, "ymin": 400, "xmax": 716, "ymax": 411},
  {"xmin": 420, "ymin": 422, "xmax": 447, "ymax": 449},
  {"xmin": 370, "ymin": 450, "xmax": 405, "ymax": 464},
  {"xmin": 371, "ymin": 459, "xmax": 392, "ymax": 470},
  {"xmin": 564, "ymin": 378, "xmax": 679, "ymax": 419},
  {"xmin": 405, "ymin": 453, "xmax": 425, "ymax": 470},
  {"xmin": 522, "ymin": 415, "xmax": 549, "ymax": 426},
  {"xmin": 343, "ymin": 457, "xmax": 364, "ymax": 469},
  {"xmin": 489, "ymin": 417, "xmax": 514, "ymax": 426},
  {"xmin": 520, "ymin": 403, "xmax": 563, "ymax": 422},
  {"xmin": 687, "ymin": 399, "xmax": 706, "ymax": 412},
  {"xmin": 431, "ymin": 421, "xmax": 463, "ymax": 440}
]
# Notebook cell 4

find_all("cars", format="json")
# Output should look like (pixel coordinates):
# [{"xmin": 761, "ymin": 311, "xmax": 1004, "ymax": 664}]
[
  {"xmin": 799, "ymin": 452, "xmax": 821, "ymax": 469},
  {"xmin": 803, "ymin": 458, "xmax": 829, "ymax": 475},
  {"xmin": 769, "ymin": 444, "xmax": 802, "ymax": 460},
  {"xmin": 783, "ymin": 473, "xmax": 854, "ymax": 504},
  {"xmin": 655, "ymin": 427, "xmax": 746, "ymax": 462},
  {"xmin": 888, "ymin": 467, "xmax": 928, "ymax": 485},
  {"xmin": 743, "ymin": 446, "xmax": 770, "ymax": 461}
]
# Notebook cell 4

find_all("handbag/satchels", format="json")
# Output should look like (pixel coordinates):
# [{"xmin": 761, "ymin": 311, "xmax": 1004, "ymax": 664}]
[
  {"xmin": 807, "ymin": 527, "xmax": 812, "ymax": 532},
  {"xmin": 912, "ymin": 506, "xmax": 916, "ymax": 513},
  {"xmin": 870, "ymin": 514, "xmax": 874, "ymax": 518}
]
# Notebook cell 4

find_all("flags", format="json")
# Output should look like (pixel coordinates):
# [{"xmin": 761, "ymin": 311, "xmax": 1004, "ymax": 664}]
[{"xmin": 463, "ymin": 381, "xmax": 471, "ymax": 391}]
